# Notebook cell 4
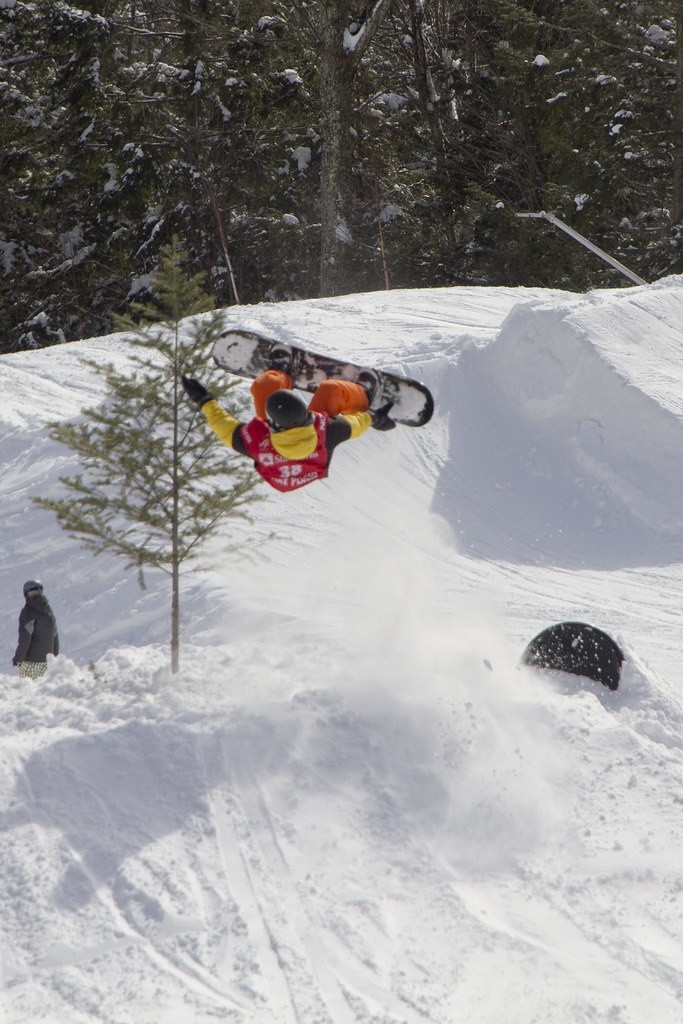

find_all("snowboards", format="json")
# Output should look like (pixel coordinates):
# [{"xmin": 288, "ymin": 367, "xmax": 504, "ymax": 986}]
[{"xmin": 208, "ymin": 328, "xmax": 434, "ymax": 427}]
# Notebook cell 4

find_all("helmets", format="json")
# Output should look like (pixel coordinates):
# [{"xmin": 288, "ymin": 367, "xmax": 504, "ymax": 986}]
[
  {"xmin": 265, "ymin": 387, "xmax": 309, "ymax": 433},
  {"xmin": 23, "ymin": 580, "xmax": 44, "ymax": 599}
]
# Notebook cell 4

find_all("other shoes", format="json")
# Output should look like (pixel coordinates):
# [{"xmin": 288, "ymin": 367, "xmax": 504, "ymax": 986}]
[
  {"xmin": 355, "ymin": 370, "xmax": 378, "ymax": 405},
  {"xmin": 270, "ymin": 343, "xmax": 292, "ymax": 375}
]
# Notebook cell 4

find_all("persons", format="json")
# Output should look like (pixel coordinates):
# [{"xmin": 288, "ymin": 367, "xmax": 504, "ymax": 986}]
[
  {"xmin": 12, "ymin": 581, "xmax": 59, "ymax": 679},
  {"xmin": 182, "ymin": 345, "xmax": 396, "ymax": 493}
]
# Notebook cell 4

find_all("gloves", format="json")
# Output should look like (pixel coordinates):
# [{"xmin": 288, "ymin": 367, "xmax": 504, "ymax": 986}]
[
  {"xmin": 181, "ymin": 374, "xmax": 214, "ymax": 409},
  {"xmin": 368, "ymin": 401, "xmax": 397, "ymax": 431},
  {"xmin": 12, "ymin": 657, "xmax": 22, "ymax": 667}
]
